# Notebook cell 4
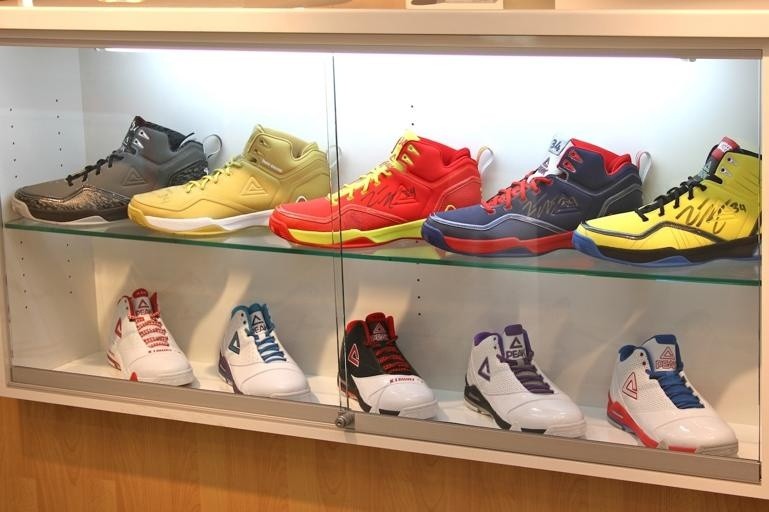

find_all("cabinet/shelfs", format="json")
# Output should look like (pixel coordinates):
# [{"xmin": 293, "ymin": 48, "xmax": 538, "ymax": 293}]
[{"xmin": 0, "ymin": 0, "xmax": 769, "ymax": 507}]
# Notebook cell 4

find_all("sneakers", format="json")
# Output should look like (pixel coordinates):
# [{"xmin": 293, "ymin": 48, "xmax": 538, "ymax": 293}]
[
  {"xmin": 463, "ymin": 322, "xmax": 587, "ymax": 438},
  {"xmin": 107, "ymin": 289, "xmax": 193, "ymax": 386},
  {"xmin": 572, "ymin": 137, "xmax": 761, "ymax": 269},
  {"xmin": 338, "ymin": 313, "xmax": 439, "ymax": 420},
  {"xmin": 270, "ymin": 129, "xmax": 494, "ymax": 248},
  {"xmin": 11, "ymin": 116, "xmax": 223, "ymax": 228},
  {"xmin": 420, "ymin": 138, "xmax": 651, "ymax": 257},
  {"xmin": 606, "ymin": 333, "xmax": 738, "ymax": 456},
  {"xmin": 127, "ymin": 125, "xmax": 333, "ymax": 238},
  {"xmin": 218, "ymin": 303, "xmax": 311, "ymax": 398}
]
